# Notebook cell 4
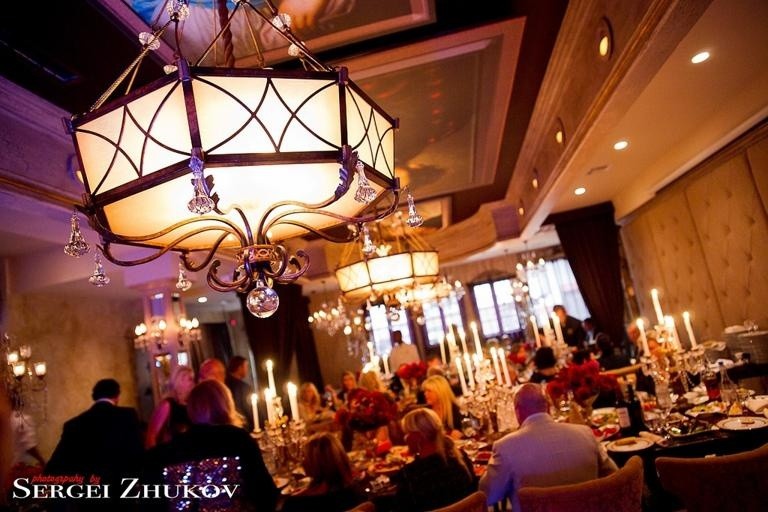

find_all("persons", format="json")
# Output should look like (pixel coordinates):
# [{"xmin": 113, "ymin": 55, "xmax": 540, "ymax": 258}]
[
  {"xmin": 225, "ymin": 354, "xmax": 268, "ymax": 433},
  {"xmin": 281, "ymin": 432, "xmax": 372, "ymax": 511},
  {"xmin": 136, "ymin": 378, "xmax": 282, "ymax": 511},
  {"xmin": 395, "ymin": 407, "xmax": 479, "ymax": 512},
  {"xmin": 477, "ymin": 382, "xmax": 652, "ymax": 512},
  {"xmin": 144, "ymin": 365, "xmax": 196, "ymax": 450},
  {"xmin": 42, "ymin": 378, "xmax": 144, "ymax": 483},
  {"xmin": 297, "ymin": 304, "xmax": 630, "ymax": 453},
  {"xmin": 198, "ymin": 358, "xmax": 226, "ymax": 385},
  {"xmin": 9, "ymin": 377, "xmax": 46, "ymax": 476}
]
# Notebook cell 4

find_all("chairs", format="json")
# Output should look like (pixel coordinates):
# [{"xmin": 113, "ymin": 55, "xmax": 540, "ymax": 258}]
[
  {"xmin": 429, "ymin": 490, "xmax": 488, "ymax": 512},
  {"xmin": 518, "ymin": 455, "xmax": 644, "ymax": 512},
  {"xmin": 347, "ymin": 500, "xmax": 374, "ymax": 512},
  {"xmin": 655, "ymin": 444, "xmax": 767, "ymax": 511}
]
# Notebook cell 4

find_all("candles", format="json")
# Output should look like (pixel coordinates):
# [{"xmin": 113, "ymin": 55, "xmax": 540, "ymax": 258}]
[
  {"xmin": 636, "ymin": 319, "xmax": 651, "ymax": 357},
  {"xmin": 362, "ymin": 342, "xmax": 392, "ymax": 378},
  {"xmin": 650, "ymin": 288, "xmax": 665, "ymax": 327},
  {"xmin": 437, "ymin": 321, "xmax": 512, "ymax": 396},
  {"xmin": 525, "ymin": 305, "xmax": 567, "ymax": 346},
  {"xmin": 665, "ymin": 315, "xmax": 683, "ymax": 351},
  {"xmin": 251, "ymin": 359, "xmax": 299, "ymax": 433},
  {"xmin": 682, "ymin": 311, "xmax": 698, "ymax": 348}
]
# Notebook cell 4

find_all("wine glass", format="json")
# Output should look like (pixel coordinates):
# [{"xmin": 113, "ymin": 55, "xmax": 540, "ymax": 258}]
[
  {"xmin": 460, "ymin": 419, "xmax": 479, "ymax": 441},
  {"xmin": 743, "ymin": 319, "xmax": 754, "ymax": 334},
  {"xmin": 463, "ymin": 438, "xmax": 479, "ymax": 467},
  {"xmin": 743, "ymin": 318, "xmax": 754, "ymax": 335}
]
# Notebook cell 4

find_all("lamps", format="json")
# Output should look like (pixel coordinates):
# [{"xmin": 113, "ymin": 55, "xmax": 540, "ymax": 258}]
[
  {"xmin": 389, "ymin": 280, "xmax": 462, "ymax": 322},
  {"xmin": 63, "ymin": 2, "xmax": 398, "ymax": 319},
  {"xmin": 510, "ymin": 256, "xmax": 571, "ymax": 311},
  {"xmin": 335, "ymin": 181, "xmax": 440, "ymax": 299},
  {"xmin": 302, "ymin": 287, "xmax": 374, "ymax": 341},
  {"xmin": 128, "ymin": 291, "xmax": 207, "ymax": 348},
  {"xmin": 0, "ymin": 334, "xmax": 54, "ymax": 425}
]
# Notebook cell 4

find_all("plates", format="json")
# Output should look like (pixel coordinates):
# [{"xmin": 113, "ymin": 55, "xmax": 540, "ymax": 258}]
[
  {"xmin": 606, "ymin": 437, "xmax": 655, "ymax": 453},
  {"xmin": 720, "ymin": 325, "xmax": 768, "ymax": 364},
  {"xmin": 744, "ymin": 394, "xmax": 768, "ymax": 415},
  {"xmin": 280, "ymin": 476, "xmax": 312, "ymax": 497},
  {"xmin": 737, "ymin": 388, "xmax": 756, "ymax": 401},
  {"xmin": 346, "ymin": 450, "xmax": 359, "ymax": 462},
  {"xmin": 590, "ymin": 407, "xmax": 618, "ymax": 426},
  {"xmin": 597, "ymin": 424, "xmax": 621, "ymax": 441},
  {"xmin": 685, "ymin": 406, "xmax": 721, "ymax": 417},
  {"xmin": 272, "ymin": 477, "xmax": 289, "ymax": 488},
  {"xmin": 716, "ymin": 416, "xmax": 768, "ymax": 430},
  {"xmin": 457, "ymin": 441, "xmax": 487, "ymax": 450}
]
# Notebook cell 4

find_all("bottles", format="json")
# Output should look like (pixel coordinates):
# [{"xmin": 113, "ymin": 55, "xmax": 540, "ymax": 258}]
[
  {"xmin": 701, "ymin": 364, "xmax": 722, "ymax": 401},
  {"xmin": 615, "ymin": 387, "xmax": 631, "ymax": 436},
  {"xmin": 567, "ymin": 391, "xmax": 586, "ymax": 424},
  {"xmin": 718, "ymin": 361, "xmax": 741, "ymax": 416},
  {"xmin": 626, "ymin": 383, "xmax": 646, "ymax": 435}
]
